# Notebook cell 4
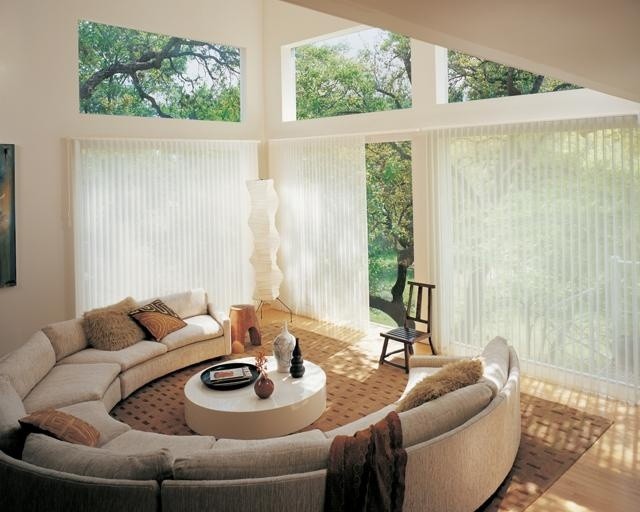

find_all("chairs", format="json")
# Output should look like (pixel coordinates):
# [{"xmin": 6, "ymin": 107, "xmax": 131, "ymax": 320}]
[{"xmin": 380, "ymin": 280, "xmax": 437, "ymax": 374}]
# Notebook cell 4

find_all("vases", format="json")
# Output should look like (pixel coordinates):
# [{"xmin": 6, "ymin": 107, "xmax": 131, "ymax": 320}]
[
  {"xmin": 254, "ymin": 376, "xmax": 274, "ymax": 399},
  {"xmin": 290, "ymin": 337, "xmax": 306, "ymax": 378},
  {"xmin": 271, "ymin": 320, "xmax": 294, "ymax": 373}
]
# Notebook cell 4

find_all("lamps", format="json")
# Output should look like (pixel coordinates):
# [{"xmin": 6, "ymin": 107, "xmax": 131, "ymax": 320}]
[{"xmin": 246, "ymin": 178, "xmax": 294, "ymax": 323}]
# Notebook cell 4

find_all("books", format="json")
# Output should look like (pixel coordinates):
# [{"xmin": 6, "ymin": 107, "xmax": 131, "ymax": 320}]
[{"xmin": 208, "ymin": 365, "xmax": 252, "ymax": 383}]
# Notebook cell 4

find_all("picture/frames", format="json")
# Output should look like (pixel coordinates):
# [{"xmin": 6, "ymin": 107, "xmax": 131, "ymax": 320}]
[{"xmin": 0, "ymin": 143, "xmax": 17, "ymax": 289}]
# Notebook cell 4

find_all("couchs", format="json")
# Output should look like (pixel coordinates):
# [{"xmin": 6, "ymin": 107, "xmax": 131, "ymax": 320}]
[{"xmin": 0, "ymin": 287, "xmax": 522, "ymax": 512}]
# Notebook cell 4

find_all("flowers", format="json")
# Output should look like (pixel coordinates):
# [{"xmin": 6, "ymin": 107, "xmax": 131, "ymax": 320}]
[{"xmin": 251, "ymin": 349, "xmax": 268, "ymax": 376}]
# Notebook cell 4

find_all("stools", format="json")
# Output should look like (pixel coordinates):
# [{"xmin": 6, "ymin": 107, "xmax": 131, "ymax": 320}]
[{"xmin": 227, "ymin": 303, "xmax": 262, "ymax": 347}]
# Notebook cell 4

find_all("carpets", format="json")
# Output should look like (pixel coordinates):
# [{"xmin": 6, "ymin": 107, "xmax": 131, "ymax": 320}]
[{"xmin": 107, "ymin": 320, "xmax": 616, "ymax": 512}]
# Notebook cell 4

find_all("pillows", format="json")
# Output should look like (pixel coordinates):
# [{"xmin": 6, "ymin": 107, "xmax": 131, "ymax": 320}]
[
  {"xmin": 392, "ymin": 356, "xmax": 484, "ymax": 414},
  {"xmin": 83, "ymin": 295, "xmax": 146, "ymax": 351},
  {"xmin": 125, "ymin": 299, "xmax": 187, "ymax": 342},
  {"xmin": 17, "ymin": 405, "xmax": 102, "ymax": 448}
]
